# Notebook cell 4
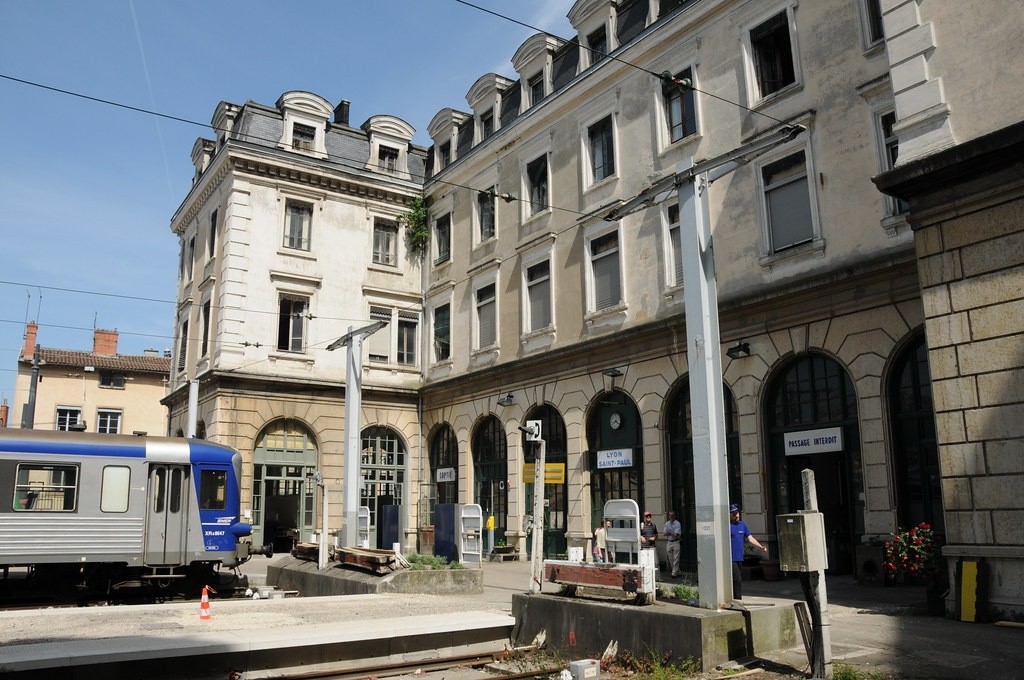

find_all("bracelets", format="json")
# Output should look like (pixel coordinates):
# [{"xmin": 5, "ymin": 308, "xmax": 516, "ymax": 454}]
[
  {"xmin": 667, "ymin": 531, "xmax": 668, "ymax": 533},
  {"xmin": 669, "ymin": 532, "xmax": 670, "ymax": 535}
]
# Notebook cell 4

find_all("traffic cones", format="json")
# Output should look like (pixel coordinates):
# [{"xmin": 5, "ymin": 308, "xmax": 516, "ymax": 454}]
[{"xmin": 198, "ymin": 587, "xmax": 212, "ymax": 620}]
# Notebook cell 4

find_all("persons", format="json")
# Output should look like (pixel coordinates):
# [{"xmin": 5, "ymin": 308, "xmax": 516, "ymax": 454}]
[
  {"xmin": 663, "ymin": 511, "xmax": 682, "ymax": 578},
  {"xmin": 730, "ymin": 504, "xmax": 767, "ymax": 599},
  {"xmin": 593, "ymin": 520, "xmax": 613, "ymax": 562},
  {"xmin": 640, "ymin": 512, "xmax": 664, "ymax": 581}
]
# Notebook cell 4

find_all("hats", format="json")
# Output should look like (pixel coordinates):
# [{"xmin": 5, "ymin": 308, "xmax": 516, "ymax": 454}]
[
  {"xmin": 730, "ymin": 504, "xmax": 739, "ymax": 513},
  {"xmin": 644, "ymin": 511, "xmax": 652, "ymax": 516}
]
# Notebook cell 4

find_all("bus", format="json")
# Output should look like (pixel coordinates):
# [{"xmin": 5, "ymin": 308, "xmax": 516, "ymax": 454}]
[{"xmin": 0, "ymin": 424, "xmax": 276, "ymax": 604}]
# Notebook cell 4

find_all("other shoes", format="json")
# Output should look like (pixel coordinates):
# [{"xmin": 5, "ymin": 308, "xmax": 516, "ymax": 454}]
[
  {"xmin": 659, "ymin": 578, "xmax": 664, "ymax": 582},
  {"xmin": 672, "ymin": 574, "xmax": 682, "ymax": 579}
]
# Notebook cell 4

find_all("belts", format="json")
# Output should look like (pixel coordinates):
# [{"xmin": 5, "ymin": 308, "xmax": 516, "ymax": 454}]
[{"xmin": 670, "ymin": 539, "xmax": 678, "ymax": 542}]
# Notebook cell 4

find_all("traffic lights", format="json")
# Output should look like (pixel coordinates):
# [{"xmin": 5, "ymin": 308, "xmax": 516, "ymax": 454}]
[{"xmin": 518, "ymin": 420, "xmax": 543, "ymax": 441}]
